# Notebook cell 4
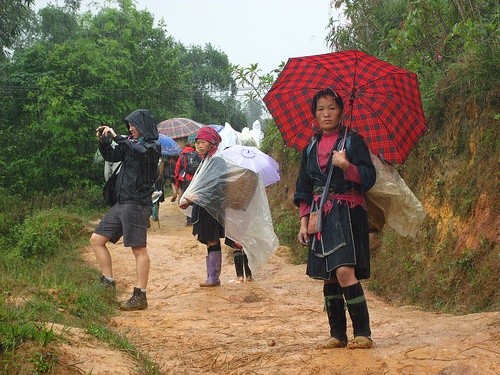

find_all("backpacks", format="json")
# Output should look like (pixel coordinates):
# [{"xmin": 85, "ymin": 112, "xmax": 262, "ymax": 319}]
[{"xmin": 181, "ymin": 152, "xmax": 202, "ymax": 174}]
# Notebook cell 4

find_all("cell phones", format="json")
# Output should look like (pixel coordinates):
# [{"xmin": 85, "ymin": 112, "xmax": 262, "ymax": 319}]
[{"xmin": 98, "ymin": 128, "xmax": 104, "ymax": 136}]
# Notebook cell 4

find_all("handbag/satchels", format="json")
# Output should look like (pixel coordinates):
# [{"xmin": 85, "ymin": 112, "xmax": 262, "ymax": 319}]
[
  {"xmin": 102, "ymin": 174, "xmax": 117, "ymax": 207},
  {"xmin": 307, "ymin": 209, "xmax": 322, "ymax": 235}
]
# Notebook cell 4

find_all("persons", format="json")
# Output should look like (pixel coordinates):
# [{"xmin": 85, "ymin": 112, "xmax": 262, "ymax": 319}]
[
  {"xmin": 178, "ymin": 126, "xmax": 227, "ymax": 287},
  {"xmin": 149, "ymin": 156, "xmax": 167, "ymax": 221},
  {"xmin": 293, "ymin": 88, "xmax": 377, "ymax": 349},
  {"xmin": 103, "ymin": 143, "xmax": 123, "ymax": 182},
  {"xmin": 169, "ymin": 157, "xmax": 183, "ymax": 205},
  {"xmin": 233, "ymin": 242, "xmax": 254, "ymax": 283},
  {"xmin": 175, "ymin": 142, "xmax": 203, "ymax": 227},
  {"xmin": 90, "ymin": 109, "xmax": 161, "ymax": 311}
]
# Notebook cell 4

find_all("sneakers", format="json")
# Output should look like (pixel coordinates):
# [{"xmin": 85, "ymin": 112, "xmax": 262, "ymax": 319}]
[
  {"xmin": 100, "ymin": 275, "xmax": 116, "ymax": 291},
  {"xmin": 121, "ymin": 287, "xmax": 148, "ymax": 311}
]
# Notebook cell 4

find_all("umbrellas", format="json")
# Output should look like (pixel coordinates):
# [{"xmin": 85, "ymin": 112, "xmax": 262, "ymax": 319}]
[
  {"xmin": 209, "ymin": 125, "xmax": 224, "ymax": 133},
  {"xmin": 264, "ymin": 50, "xmax": 429, "ymax": 166},
  {"xmin": 157, "ymin": 133, "xmax": 182, "ymax": 156},
  {"xmin": 219, "ymin": 145, "xmax": 281, "ymax": 187},
  {"xmin": 156, "ymin": 118, "xmax": 203, "ymax": 139}
]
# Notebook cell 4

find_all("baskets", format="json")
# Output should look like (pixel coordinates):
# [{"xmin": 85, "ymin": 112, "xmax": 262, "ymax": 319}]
[
  {"xmin": 223, "ymin": 162, "xmax": 258, "ymax": 212},
  {"xmin": 366, "ymin": 158, "xmax": 399, "ymax": 233}
]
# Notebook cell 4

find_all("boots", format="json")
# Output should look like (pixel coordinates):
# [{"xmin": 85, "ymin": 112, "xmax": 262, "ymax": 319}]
[{"xmin": 199, "ymin": 251, "xmax": 222, "ymax": 287}]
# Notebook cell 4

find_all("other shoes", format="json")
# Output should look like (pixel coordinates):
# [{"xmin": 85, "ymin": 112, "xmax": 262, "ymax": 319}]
[
  {"xmin": 347, "ymin": 336, "xmax": 373, "ymax": 349},
  {"xmin": 229, "ymin": 277, "xmax": 244, "ymax": 284},
  {"xmin": 321, "ymin": 337, "xmax": 347, "ymax": 348},
  {"xmin": 246, "ymin": 276, "xmax": 254, "ymax": 283}
]
